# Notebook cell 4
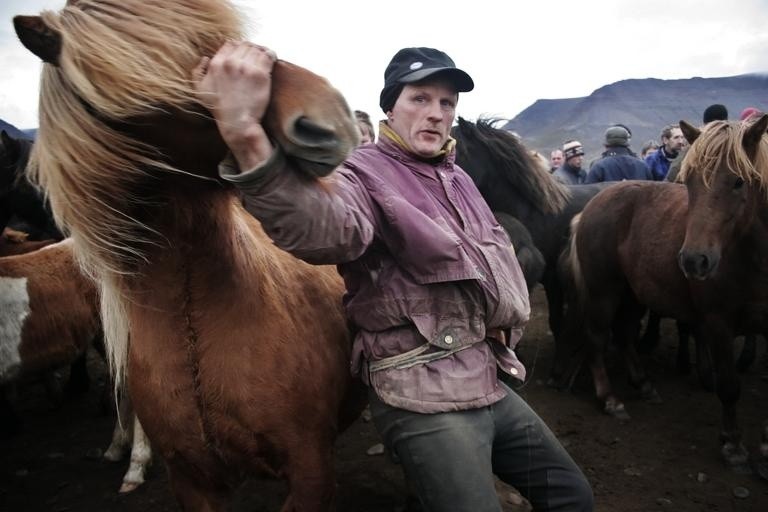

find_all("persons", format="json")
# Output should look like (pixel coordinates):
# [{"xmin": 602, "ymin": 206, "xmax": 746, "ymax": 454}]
[
  {"xmin": 353, "ymin": 110, "xmax": 377, "ymax": 147},
  {"xmin": 189, "ymin": 36, "xmax": 601, "ymax": 511},
  {"xmin": 496, "ymin": 101, "xmax": 767, "ymax": 189}
]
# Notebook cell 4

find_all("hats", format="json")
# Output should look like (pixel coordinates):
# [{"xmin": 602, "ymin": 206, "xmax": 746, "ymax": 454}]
[
  {"xmin": 702, "ymin": 104, "xmax": 727, "ymax": 123},
  {"xmin": 603, "ymin": 126, "xmax": 630, "ymax": 147},
  {"xmin": 379, "ymin": 47, "xmax": 473, "ymax": 109},
  {"xmin": 563, "ymin": 139, "xmax": 583, "ymax": 159}
]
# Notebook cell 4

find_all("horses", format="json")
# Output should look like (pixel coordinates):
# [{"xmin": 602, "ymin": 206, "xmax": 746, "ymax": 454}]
[{"xmin": 0, "ymin": 0, "xmax": 768, "ymax": 512}]
